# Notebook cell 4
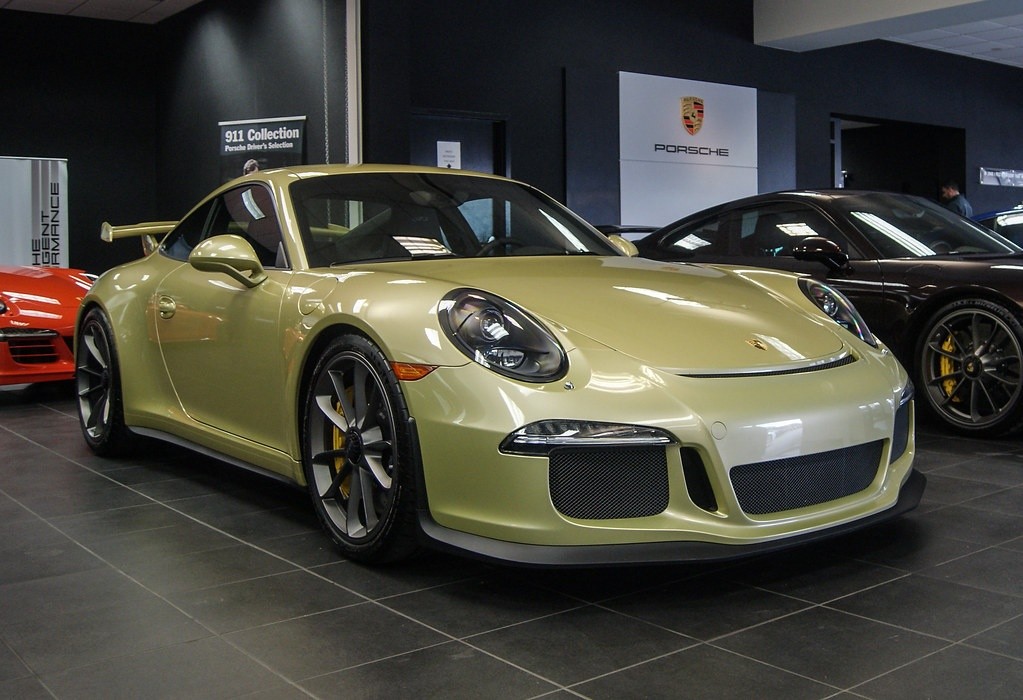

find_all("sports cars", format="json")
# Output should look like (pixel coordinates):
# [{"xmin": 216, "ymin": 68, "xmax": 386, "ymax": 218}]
[
  {"xmin": 0, "ymin": 265, "xmax": 108, "ymax": 385},
  {"xmin": 83, "ymin": 166, "xmax": 931, "ymax": 572}
]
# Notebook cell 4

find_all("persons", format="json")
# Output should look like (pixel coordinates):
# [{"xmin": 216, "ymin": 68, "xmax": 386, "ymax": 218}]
[{"xmin": 940, "ymin": 179, "xmax": 973, "ymax": 219}]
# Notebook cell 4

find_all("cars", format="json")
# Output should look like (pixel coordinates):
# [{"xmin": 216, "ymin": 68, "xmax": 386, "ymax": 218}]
[
  {"xmin": 922, "ymin": 209, "xmax": 1023, "ymax": 252},
  {"xmin": 597, "ymin": 188, "xmax": 1022, "ymax": 441}
]
